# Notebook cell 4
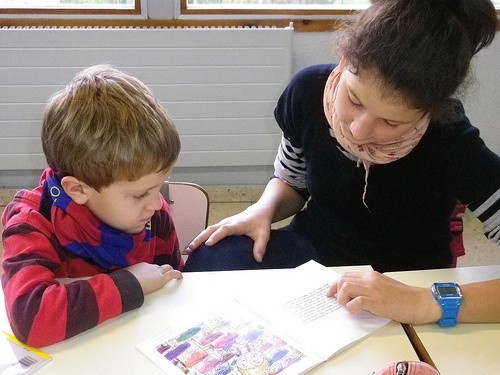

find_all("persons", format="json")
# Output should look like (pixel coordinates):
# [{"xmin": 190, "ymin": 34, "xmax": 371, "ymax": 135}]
[
  {"xmin": 1, "ymin": 64, "xmax": 186, "ymax": 346},
  {"xmin": 184, "ymin": 0, "xmax": 500, "ymax": 328}
]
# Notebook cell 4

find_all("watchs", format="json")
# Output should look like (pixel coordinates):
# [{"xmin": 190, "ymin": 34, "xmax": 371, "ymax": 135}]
[{"xmin": 430, "ymin": 282, "xmax": 462, "ymax": 327}]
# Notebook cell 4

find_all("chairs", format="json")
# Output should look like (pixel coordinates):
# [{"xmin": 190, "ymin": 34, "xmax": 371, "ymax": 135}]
[{"xmin": 158, "ymin": 179, "xmax": 208, "ymax": 271}]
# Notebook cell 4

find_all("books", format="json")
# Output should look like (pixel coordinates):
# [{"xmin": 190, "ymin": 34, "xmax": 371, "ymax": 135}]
[
  {"xmin": 1, "ymin": 329, "xmax": 54, "ymax": 375},
  {"xmin": 135, "ymin": 259, "xmax": 396, "ymax": 375}
]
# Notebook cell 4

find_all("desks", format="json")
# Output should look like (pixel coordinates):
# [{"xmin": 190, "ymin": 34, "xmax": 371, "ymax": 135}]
[{"xmin": 0, "ymin": 268, "xmax": 500, "ymax": 375}]
